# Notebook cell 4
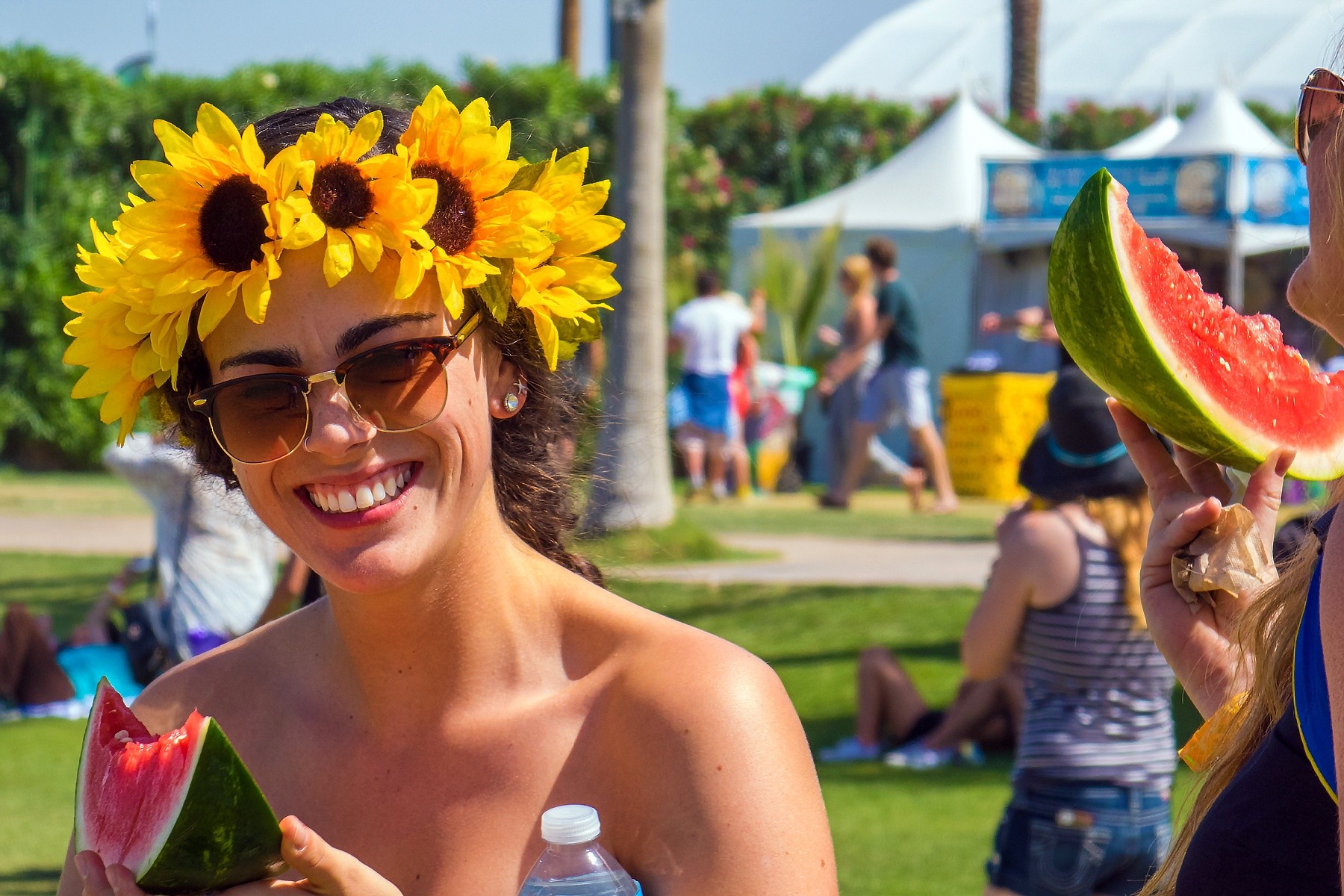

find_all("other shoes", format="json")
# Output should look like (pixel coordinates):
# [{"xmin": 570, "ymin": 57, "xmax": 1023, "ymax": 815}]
[
  {"xmin": 818, "ymin": 740, "xmax": 881, "ymax": 765},
  {"xmin": 885, "ymin": 741, "xmax": 958, "ymax": 769}
]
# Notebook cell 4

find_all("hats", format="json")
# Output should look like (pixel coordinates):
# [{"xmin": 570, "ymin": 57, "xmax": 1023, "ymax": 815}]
[
  {"xmin": 1019, "ymin": 369, "xmax": 1142, "ymax": 499},
  {"xmin": 838, "ymin": 252, "xmax": 871, "ymax": 285}
]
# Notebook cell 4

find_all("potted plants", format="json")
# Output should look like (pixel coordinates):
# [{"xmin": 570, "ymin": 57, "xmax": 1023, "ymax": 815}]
[{"xmin": 743, "ymin": 216, "xmax": 852, "ymax": 483}]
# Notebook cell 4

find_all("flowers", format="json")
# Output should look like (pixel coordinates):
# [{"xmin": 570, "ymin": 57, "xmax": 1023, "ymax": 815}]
[{"xmin": 61, "ymin": 84, "xmax": 626, "ymax": 450}]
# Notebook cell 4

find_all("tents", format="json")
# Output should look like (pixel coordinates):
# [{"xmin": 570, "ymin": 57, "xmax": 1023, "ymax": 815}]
[
  {"xmin": 972, "ymin": 87, "xmax": 1307, "ymax": 339},
  {"xmin": 729, "ymin": 93, "xmax": 1054, "ymax": 468}
]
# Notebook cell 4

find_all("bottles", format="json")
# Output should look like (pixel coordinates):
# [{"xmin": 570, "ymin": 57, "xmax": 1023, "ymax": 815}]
[{"xmin": 518, "ymin": 805, "xmax": 643, "ymax": 896}]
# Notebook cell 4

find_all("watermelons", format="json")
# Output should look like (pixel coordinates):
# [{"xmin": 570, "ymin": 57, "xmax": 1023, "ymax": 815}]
[
  {"xmin": 75, "ymin": 676, "xmax": 291, "ymax": 895},
  {"xmin": 1046, "ymin": 166, "xmax": 1344, "ymax": 484}
]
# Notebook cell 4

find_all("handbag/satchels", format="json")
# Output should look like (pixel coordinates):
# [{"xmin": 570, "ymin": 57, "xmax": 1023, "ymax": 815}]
[{"xmin": 121, "ymin": 602, "xmax": 194, "ymax": 686}]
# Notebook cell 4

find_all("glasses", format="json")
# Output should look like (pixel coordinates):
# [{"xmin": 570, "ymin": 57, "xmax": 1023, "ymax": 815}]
[
  {"xmin": 1296, "ymin": 68, "xmax": 1344, "ymax": 164},
  {"xmin": 188, "ymin": 310, "xmax": 482, "ymax": 464}
]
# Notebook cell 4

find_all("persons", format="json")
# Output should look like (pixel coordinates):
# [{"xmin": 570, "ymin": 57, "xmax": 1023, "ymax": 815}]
[
  {"xmin": 963, "ymin": 367, "xmax": 1179, "ymax": 896},
  {"xmin": 1102, "ymin": 69, "xmax": 1344, "ymax": 895},
  {"xmin": 667, "ymin": 273, "xmax": 769, "ymax": 503},
  {"xmin": 54, "ymin": 96, "xmax": 840, "ymax": 896},
  {"xmin": 101, "ymin": 431, "xmax": 307, "ymax": 662},
  {"xmin": 824, "ymin": 647, "xmax": 1010, "ymax": 766},
  {"xmin": 812, "ymin": 236, "xmax": 958, "ymax": 515},
  {"xmin": 551, "ymin": 340, "xmax": 602, "ymax": 472}
]
